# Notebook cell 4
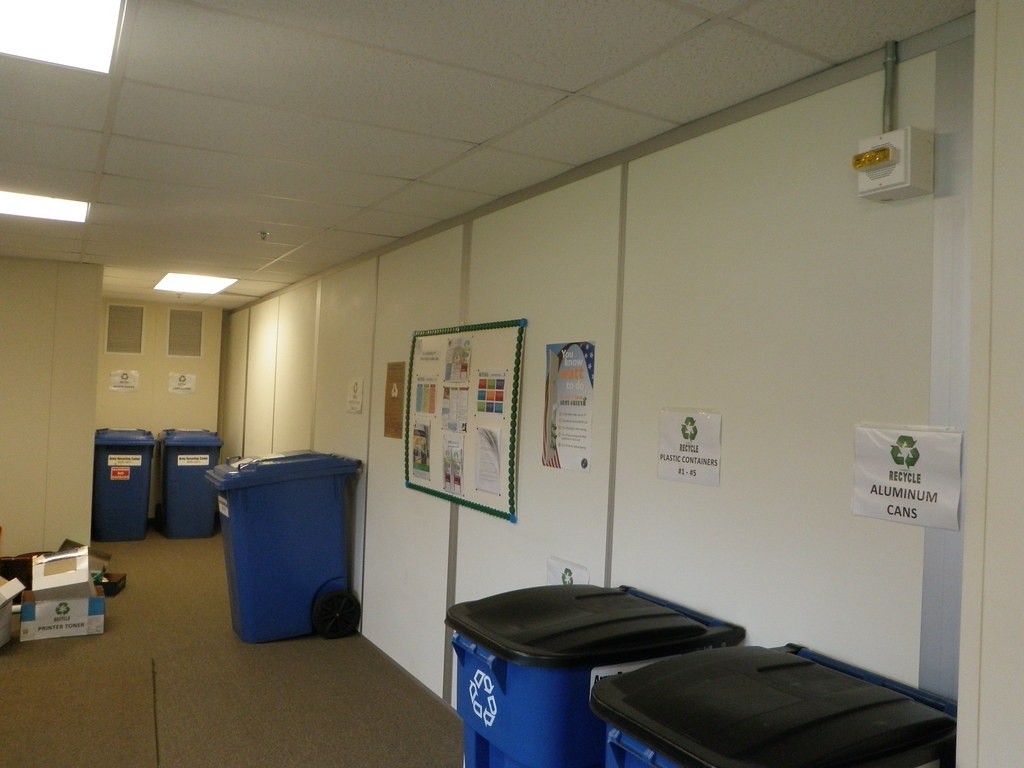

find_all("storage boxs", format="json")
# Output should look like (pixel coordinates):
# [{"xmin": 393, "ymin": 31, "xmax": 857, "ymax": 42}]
[
  {"xmin": 20, "ymin": 585, "xmax": 105, "ymax": 642},
  {"xmin": 0, "ymin": 576, "xmax": 13, "ymax": 647},
  {"xmin": 56, "ymin": 538, "xmax": 112, "ymax": 575},
  {"xmin": 0, "ymin": 577, "xmax": 26, "ymax": 608},
  {"xmin": 95, "ymin": 572, "xmax": 127, "ymax": 597},
  {"xmin": 32, "ymin": 546, "xmax": 97, "ymax": 600}
]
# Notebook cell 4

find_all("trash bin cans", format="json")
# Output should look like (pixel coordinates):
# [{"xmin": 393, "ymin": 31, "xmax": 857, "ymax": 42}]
[
  {"xmin": 443, "ymin": 584, "xmax": 748, "ymax": 768},
  {"xmin": 590, "ymin": 644, "xmax": 957, "ymax": 768},
  {"xmin": 203, "ymin": 450, "xmax": 363, "ymax": 645},
  {"xmin": 158, "ymin": 428, "xmax": 224, "ymax": 541},
  {"xmin": 91, "ymin": 428, "xmax": 156, "ymax": 544}
]
